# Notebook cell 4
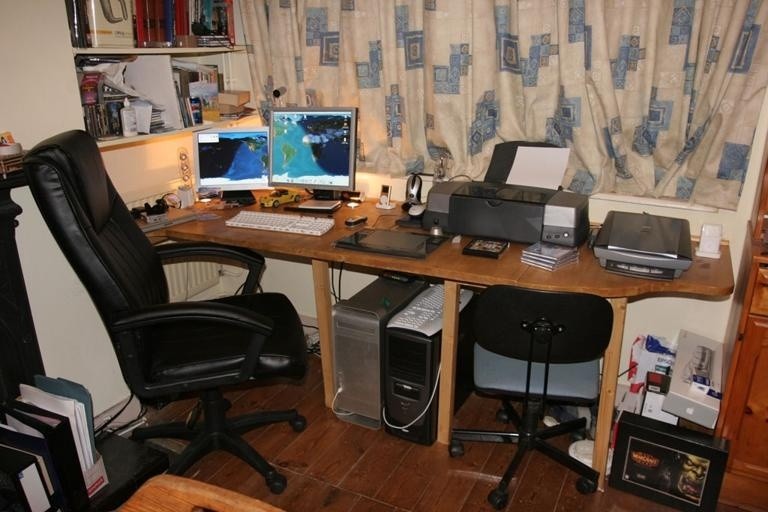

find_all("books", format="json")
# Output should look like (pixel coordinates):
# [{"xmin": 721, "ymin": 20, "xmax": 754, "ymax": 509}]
[
  {"xmin": 84, "ymin": 101, "xmax": 166, "ymax": 138},
  {"xmin": 519, "ymin": 241, "xmax": 577, "ymax": 273},
  {"xmin": 172, "ymin": 57, "xmax": 226, "ymax": 127},
  {"xmin": 64, "ymin": 1, "xmax": 236, "ymax": 48},
  {"xmin": 133, "ymin": 205, "xmax": 195, "ymax": 234}
]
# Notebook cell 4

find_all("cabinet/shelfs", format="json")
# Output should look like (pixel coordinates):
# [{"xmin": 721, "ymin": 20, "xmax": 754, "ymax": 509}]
[
  {"xmin": 0, "ymin": 183, "xmax": 169, "ymax": 512},
  {"xmin": 65, "ymin": 0, "xmax": 258, "ymax": 152},
  {"xmin": 713, "ymin": 130, "xmax": 768, "ymax": 512}
]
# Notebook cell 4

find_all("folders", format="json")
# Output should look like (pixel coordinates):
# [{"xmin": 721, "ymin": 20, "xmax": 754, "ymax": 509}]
[{"xmin": 1, "ymin": 374, "xmax": 97, "ymax": 511}]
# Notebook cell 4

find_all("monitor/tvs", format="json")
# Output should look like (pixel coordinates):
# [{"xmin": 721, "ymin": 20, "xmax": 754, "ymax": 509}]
[
  {"xmin": 268, "ymin": 106, "xmax": 359, "ymax": 214},
  {"xmin": 195, "ymin": 127, "xmax": 270, "ymax": 207}
]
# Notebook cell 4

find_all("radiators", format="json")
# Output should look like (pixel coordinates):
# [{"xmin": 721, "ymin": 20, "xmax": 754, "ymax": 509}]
[{"xmin": 163, "ymin": 262, "xmax": 223, "ymax": 304}]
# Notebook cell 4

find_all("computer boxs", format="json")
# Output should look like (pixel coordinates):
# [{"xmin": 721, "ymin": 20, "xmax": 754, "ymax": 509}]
[
  {"xmin": 383, "ymin": 292, "xmax": 475, "ymax": 446},
  {"xmin": 331, "ymin": 275, "xmax": 430, "ymax": 433}
]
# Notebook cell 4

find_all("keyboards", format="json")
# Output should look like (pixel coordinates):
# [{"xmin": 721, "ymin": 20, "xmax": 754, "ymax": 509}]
[
  {"xmin": 386, "ymin": 284, "xmax": 473, "ymax": 337},
  {"xmin": 225, "ymin": 210, "xmax": 335, "ymax": 236}
]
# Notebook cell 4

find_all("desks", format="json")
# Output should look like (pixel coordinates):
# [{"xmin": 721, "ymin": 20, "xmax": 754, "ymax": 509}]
[{"xmin": 164, "ymin": 189, "xmax": 736, "ymax": 492}]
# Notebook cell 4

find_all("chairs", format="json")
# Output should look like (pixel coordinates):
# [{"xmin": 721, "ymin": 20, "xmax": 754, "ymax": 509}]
[
  {"xmin": 24, "ymin": 130, "xmax": 308, "ymax": 496},
  {"xmin": 449, "ymin": 285, "xmax": 614, "ymax": 509}
]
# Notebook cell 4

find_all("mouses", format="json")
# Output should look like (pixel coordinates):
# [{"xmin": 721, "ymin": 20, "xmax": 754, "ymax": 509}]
[
  {"xmin": 345, "ymin": 214, "xmax": 368, "ymax": 225},
  {"xmin": 406, "ymin": 175, "xmax": 423, "ymax": 205}
]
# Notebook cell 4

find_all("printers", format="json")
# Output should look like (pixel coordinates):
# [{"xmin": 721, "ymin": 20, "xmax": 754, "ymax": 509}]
[
  {"xmin": 591, "ymin": 210, "xmax": 693, "ymax": 281},
  {"xmin": 422, "ymin": 140, "xmax": 590, "ymax": 248}
]
